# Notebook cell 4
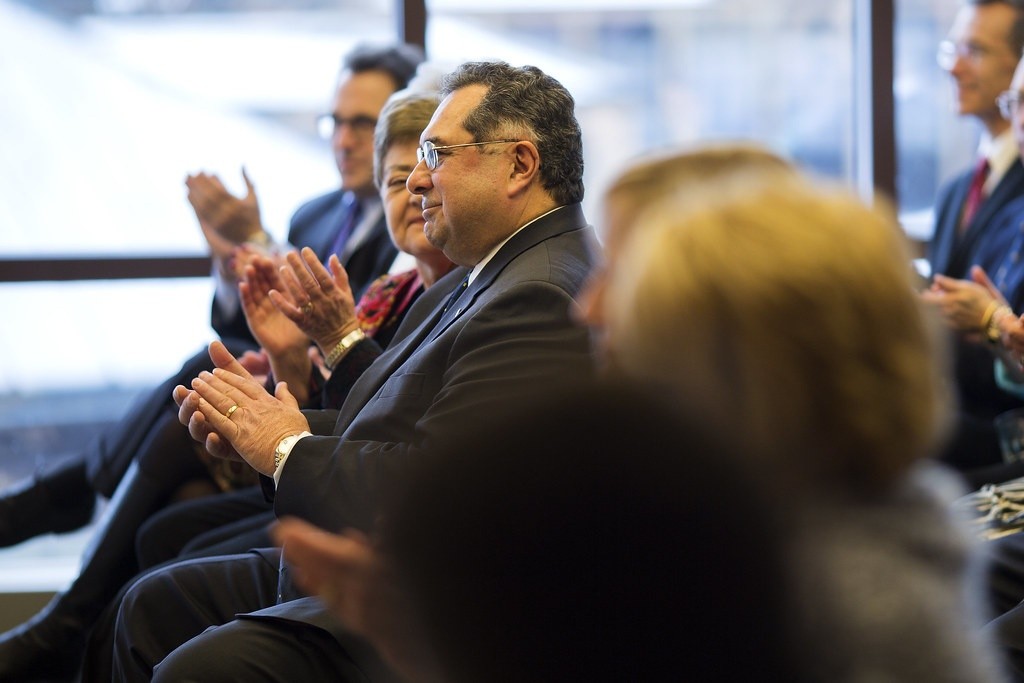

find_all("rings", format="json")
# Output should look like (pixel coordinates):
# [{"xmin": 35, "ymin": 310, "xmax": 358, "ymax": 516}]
[
  {"xmin": 300, "ymin": 303, "xmax": 315, "ymax": 315},
  {"xmin": 227, "ymin": 405, "xmax": 239, "ymax": 418}
]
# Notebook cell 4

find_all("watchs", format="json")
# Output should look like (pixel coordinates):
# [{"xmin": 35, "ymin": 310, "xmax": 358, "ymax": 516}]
[{"xmin": 275, "ymin": 435, "xmax": 302, "ymax": 475}]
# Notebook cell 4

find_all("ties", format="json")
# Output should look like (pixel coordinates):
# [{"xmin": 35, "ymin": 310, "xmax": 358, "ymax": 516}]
[
  {"xmin": 957, "ymin": 158, "xmax": 989, "ymax": 245},
  {"xmin": 326, "ymin": 195, "xmax": 366, "ymax": 272},
  {"xmin": 440, "ymin": 274, "xmax": 469, "ymax": 323}
]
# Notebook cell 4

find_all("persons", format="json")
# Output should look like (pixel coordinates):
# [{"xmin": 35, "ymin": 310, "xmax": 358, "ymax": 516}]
[
  {"xmin": 913, "ymin": 0, "xmax": 1024, "ymax": 471},
  {"xmin": 600, "ymin": 174, "xmax": 1014, "ymax": 682},
  {"xmin": 81, "ymin": 89, "xmax": 452, "ymax": 683},
  {"xmin": 562, "ymin": 145, "xmax": 805, "ymax": 375},
  {"xmin": 360, "ymin": 367, "xmax": 812, "ymax": 681},
  {"xmin": 918, "ymin": 52, "xmax": 1024, "ymax": 388},
  {"xmin": 109, "ymin": 60, "xmax": 612, "ymax": 683},
  {"xmin": 134, "ymin": 63, "xmax": 464, "ymax": 565},
  {"xmin": 944, "ymin": 530, "xmax": 1024, "ymax": 683},
  {"xmin": 3, "ymin": 40, "xmax": 426, "ymax": 683}
]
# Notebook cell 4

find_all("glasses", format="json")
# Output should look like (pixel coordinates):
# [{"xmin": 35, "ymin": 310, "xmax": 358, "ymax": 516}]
[
  {"xmin": 995, "ymin": 89, "xmax": 1024, "ymax": 121},
  {"xmin": 933, "ymin": 42, "xmax": 1014, "ymax": 66},
  {"xmin": 416, "ymin": 137, "xmax": 523, "ymax": 170},
  {"xmin": 315, "ymin": 114, "xmax": 377, "ymax": 138}
]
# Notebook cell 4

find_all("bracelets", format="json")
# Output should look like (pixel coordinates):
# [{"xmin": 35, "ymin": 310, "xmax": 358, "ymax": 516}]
[
  {"xmin": 975, "ymin": 301, "xmax": 1014, "ymax": 347},
  {"xmin": 323, "ymin": 328, "xmax": 367, "ymax": 371},
  {"xmin": 245, "ymin": 232, "xmax": 271, "ymax": 249}
]
